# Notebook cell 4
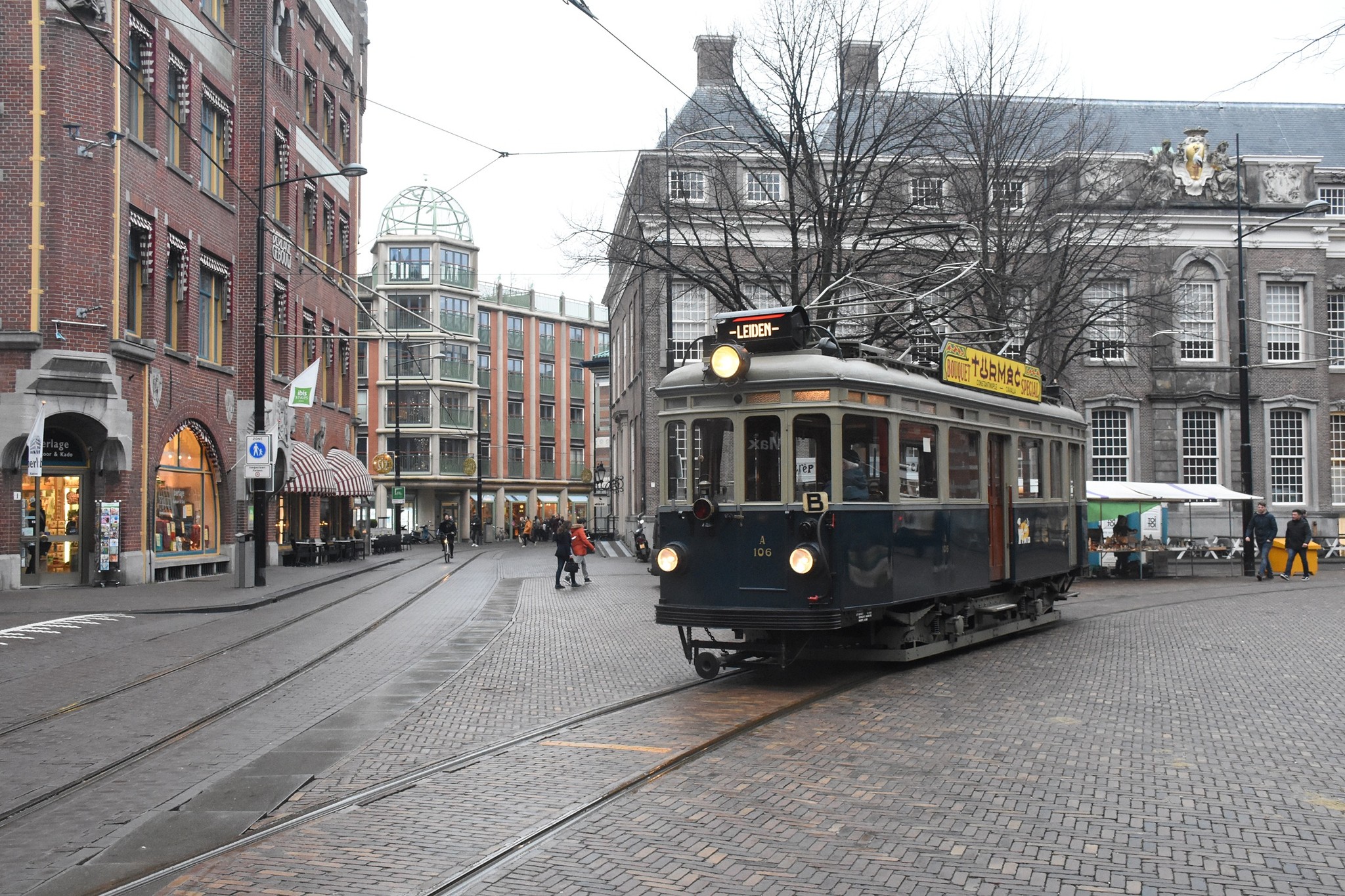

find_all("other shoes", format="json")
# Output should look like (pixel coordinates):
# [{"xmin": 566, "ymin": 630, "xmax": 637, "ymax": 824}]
[
  {"xmin": 1257, "ymin": 573, "xmax": 1261, "ymax": 581},
  {"xmin": 571, "ymin": 583, "xmax": 582, "ymax": 587},
  {"xmin": 1266, "ymin": 573, "xmax": 1274, "ymax": 579},
  {"xmin": 522, "ymin": 538, "xmax": 556, "ymax": 548},
  {"xmin": 471, "ymin": 544, "xmax": 477, "ymax": 546},
  {"xmin": 555, "ymin": 583, "xmax": 565, "ymax": 589},
  {"xmin": 564, "ymin": 576, "xmax": 571, "ymax": 585},
  {"xmin": 451, "ymin": 555, "xmax": 453, "ymax": 558},
  {"xmin": 584, "ymin": 578, "xmax": 593, "ymax": 584},
  {"xmin": 442, "ymin": 548, "xmax": 445, "ymax": 551},
  {"xmin": 475, "ymin": 545, "xmax": 479, "ymax": 547}
]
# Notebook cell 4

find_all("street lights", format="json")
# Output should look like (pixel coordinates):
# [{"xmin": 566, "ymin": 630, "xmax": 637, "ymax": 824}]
[
  {"xmin": 1237, "ymin": 197, "xmax": 1331, "ymax": 576},
  {"xmin": 393, "ymin": 352, "xmax": 447, "ymax": 551},
  {"xmin": 250, "ymin": 162, "xmax": 369, "ymax": 587}
]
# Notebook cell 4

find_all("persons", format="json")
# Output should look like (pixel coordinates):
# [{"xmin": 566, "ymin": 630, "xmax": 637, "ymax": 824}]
[
  {"xmin": 1281, "ymin": 509, "xmax": 1313, "ymax": 582},
  {"xmin": 470, "ymin": 513, "xmax": 481, "ymax": 547},
  {"xmin": 511, "ymin": 511, "xmax": 567, "ymax": 548},
  {"xmin": 564, "ymin": 517, "xmax": 595, "ymax": 586},
  {"xmin": 824, "ymin": 450, "xmax": 870, "ymax": 503},
  {"xmin": 24, "ymin": 497, "xmax": 47, "ymax": 574},
  {"xmin": 555, "ymin": 521, "xmax": 582, "ymax": 589},
  {"xmin": 438, "ymin": 513, "xmax": 457, "ymax": 558},
  {"xmin": 1113, "ymin": 515, "xmax": 1137, "ymax": 577},
  {"xmin": 1245, "ymin": 501, "xmax": 1278, "ymax": 581}
]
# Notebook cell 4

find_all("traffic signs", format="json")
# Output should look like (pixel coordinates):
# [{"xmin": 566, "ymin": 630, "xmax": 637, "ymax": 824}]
[{"xmin": 244, "ymin": 434, "xmax": 272, "ymax": 478}]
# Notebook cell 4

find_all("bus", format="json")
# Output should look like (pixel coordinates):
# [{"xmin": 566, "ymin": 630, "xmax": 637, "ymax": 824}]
[{"xmin": 649, "ymin": 304, "xmax": 1090, "ymax": 690}]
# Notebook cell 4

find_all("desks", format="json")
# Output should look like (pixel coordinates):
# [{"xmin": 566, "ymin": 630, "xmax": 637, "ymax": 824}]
[{"xmin": 1086, "ymin": 546, "xmax": 1246, "ymax": 580}]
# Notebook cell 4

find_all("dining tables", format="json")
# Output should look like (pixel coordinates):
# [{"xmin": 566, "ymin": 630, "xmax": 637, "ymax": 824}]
[{"xmin": 1165, "ymin": 532, "xmax": 1344, "ymax": 559}]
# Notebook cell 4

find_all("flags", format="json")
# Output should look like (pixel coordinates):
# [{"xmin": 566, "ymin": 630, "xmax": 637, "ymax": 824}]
[
  {"xmin": 266, "ymin": 423, "xmax": 278, "ymax": 472},
  {"xmin": 288, "ymin": 359, "xmax": 320, "ymax": 408},
  {"xmin": 26, "ymin": 404, "xmax": 45, "ymax": 478}
]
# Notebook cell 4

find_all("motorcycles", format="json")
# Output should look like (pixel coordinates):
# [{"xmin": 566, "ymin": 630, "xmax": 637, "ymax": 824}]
[{"xmin": 628, "ymin": 519, "xmax": 651, "ymax": 563}]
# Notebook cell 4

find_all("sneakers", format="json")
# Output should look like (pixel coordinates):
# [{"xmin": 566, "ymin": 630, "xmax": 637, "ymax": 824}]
[
  {"xmin": 1302, "ymin": 576, "xmax": 1310, "ymax": 581},
  {"xmin": 1280, "ymin": 574, "xmax": 1290, "ymax": 580}
]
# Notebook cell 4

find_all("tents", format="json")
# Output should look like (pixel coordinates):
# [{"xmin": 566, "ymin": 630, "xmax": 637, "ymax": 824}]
[{"xmin": 1086, "ymin": 479, "xmax": 1266, "ymax": 583}]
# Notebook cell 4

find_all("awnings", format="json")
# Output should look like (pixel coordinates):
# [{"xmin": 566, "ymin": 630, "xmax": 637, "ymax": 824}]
[
  {"xmin": 280, "ymin": 441, "xmax": 337, "ymax": 496},
  {"xmin": 325, "ymin": 447, "xmax": 375, "ymax": 498}
]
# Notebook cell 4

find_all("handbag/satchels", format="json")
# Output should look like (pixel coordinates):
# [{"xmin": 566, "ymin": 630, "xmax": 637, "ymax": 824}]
[
  {"xmin": 520, "ymin": 533, "xmax": 526, "ymax": 538},
  {"xmin": 564, "ymin": 557, "xmax": 579, "ymax": 572}
]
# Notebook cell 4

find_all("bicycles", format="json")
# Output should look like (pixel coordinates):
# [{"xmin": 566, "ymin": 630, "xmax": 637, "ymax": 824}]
[
  {"xmin": 482, "ymin": 525, "xmax": 509, "ymax": 542},
  {"xmin": 416, "ymin": 521, "xmax": 451, "ymax": 545},
  {"xmin": 437, "ymin": 531, "xmax": 455, "ymax": 563}
]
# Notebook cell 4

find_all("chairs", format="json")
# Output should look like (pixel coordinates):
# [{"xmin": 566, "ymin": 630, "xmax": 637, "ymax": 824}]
[{"xmin": 283, "ymin": 532, "xmax": 412, "ymax": 568}]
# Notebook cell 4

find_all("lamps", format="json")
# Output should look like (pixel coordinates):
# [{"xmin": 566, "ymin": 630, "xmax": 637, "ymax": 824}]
[
  {"xmin": 593, "ymin": 460, "xmax": 624, "ymax": 495},
  {"xmin": 63, "ymin": 123, "xmax": 126, "ymax": 158}
]
